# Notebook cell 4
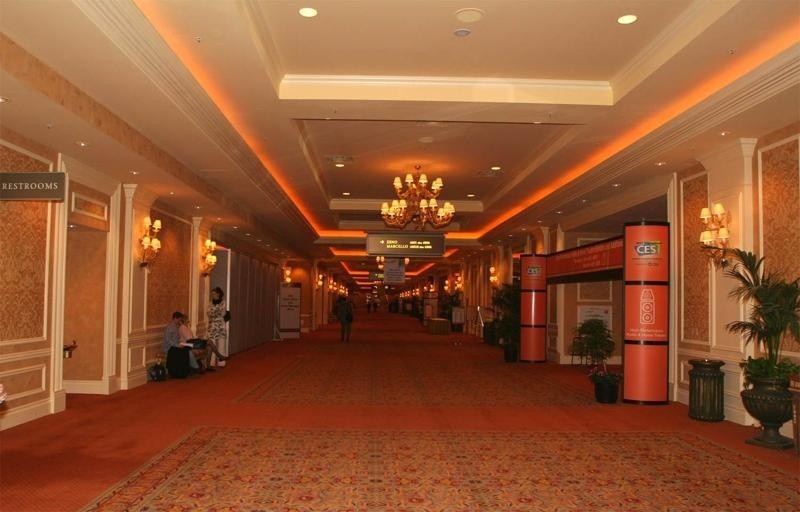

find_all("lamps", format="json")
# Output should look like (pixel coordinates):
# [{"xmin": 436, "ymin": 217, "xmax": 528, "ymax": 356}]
[
  {"xmin": 139, "ymin": 216, "xmax": 162, "ymax": 267},
  {"xmin": 381, "ymin": 164, "xmax": 456, "ymax": 231},
  {"xmin": 400, "ymin": 267, "xmax": 498, "ymax": 297},
  {"xmin": 700, "ymin": 202, "xmax": 731, "ymax": 245},
  {"xmin": 284, "ymin": 266, "xmax": 349, "ymax": 296},
  {"xmin": 201, "ymin": 238, "xmax": 218, "ymax": 276}
]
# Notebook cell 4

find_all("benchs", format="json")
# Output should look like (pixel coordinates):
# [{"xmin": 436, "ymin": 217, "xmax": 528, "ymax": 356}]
[{"xmin": 156, "ymin": 349, "xmax": 210, "ymax": 377}]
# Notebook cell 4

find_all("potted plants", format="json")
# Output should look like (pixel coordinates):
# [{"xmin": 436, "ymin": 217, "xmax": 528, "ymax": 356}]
[
  {"xmin": 491, "ymin": 284, "xmax": 521, "ymax": 362},
  {"xmin": 568, "ymin": 312, "xmax": 622, "ymax": 403},
  {"xmin": 700, "ymin": 244, "xmax": 800, "ymax": 450}
]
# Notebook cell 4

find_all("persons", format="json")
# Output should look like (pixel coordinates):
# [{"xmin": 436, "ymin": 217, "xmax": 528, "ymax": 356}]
[
  {"xmin": 338, "ymin": 296, "xmax": 353, "ymax": 343},
  {"xmin": 161, "ymin": 311, "xmax": 206, "ymax": 376},
  {"xmin": 178, "ymin": 314, "xmax": 230, "ymax": 372},
  {"xmin": 206, "ymin": 286, "xmax": 227, "ymax": 367}
]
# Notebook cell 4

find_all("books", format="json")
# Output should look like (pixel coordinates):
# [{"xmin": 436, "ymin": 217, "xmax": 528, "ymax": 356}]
[{"xmin": 181, "ymin": 342, "xmax": 195, "ymax": 350}]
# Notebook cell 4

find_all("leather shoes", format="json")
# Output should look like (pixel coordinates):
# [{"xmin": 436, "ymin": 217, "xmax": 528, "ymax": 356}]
[
  {"xmin": 219, "ymin": 356, "xmax": 231, "ymax": 361},
  {"xmin": 207, "ymin": 367, "xmax": 216, "ymax": 371}
]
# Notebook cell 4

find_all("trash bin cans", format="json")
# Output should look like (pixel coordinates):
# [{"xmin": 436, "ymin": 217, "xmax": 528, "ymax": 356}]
[
  {"xmin": 483, "ymin": 321, "xmax": 495, "ymax": 346},
  {"xmin": 688, "ymin": 359, "xmax": 725, "ymax": 421}
]
